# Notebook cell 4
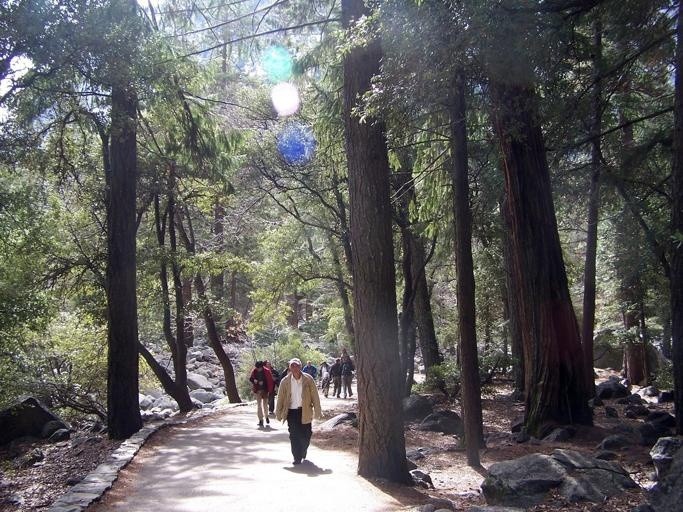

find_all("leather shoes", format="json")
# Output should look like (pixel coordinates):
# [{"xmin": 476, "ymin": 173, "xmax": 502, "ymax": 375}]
[
  {"xmin": 293, "ymin": 460, "xmax": 301, "ymax": 464},
  {"xmin": 257, "ymin": 423, "xmax": 263, "ymax": 426},
  {"xmin": 266, "ymin": 417, "xmax": 269, "ymax": 423}
]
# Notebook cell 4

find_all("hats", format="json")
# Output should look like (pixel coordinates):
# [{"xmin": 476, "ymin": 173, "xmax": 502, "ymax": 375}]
[
  {"xmin": 289, "ymin": 357, "xmax": 303, "ymax": 368},
  {"xmin": 255, "ymin": 360, "xmax": 266, "ymax": 368}
]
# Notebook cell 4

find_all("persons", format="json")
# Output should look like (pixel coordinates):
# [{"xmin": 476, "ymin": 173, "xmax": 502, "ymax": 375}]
[
  {"xmin": 264, "ymin": 361, "xmax": 281, "ymax": 414},
  {"xmin": 302, "ymin": 360, "xmax": 317, "ymax": 378},
  {"xmin": 274, "ymin": 357, "xmax": 321, "ymax": 464},
  {"xmin": 249, "ymin": 361, "xmax": 274, "ymax": 425},
  {"xmin": 280, "ymin": 360, "xmax": 290, "ymax": 380},
  {"xmin": 319, "ymin": 348, "xmax": 354, "ymax": 399}
]
